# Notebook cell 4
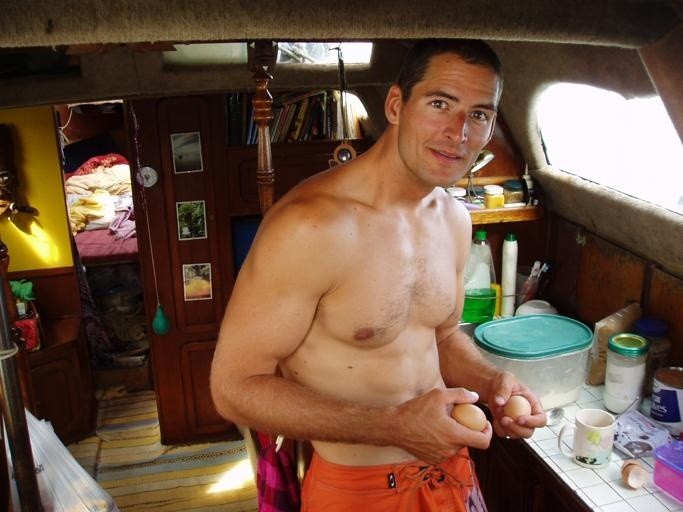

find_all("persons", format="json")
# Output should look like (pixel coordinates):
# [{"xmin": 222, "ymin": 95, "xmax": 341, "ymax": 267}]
[{"xmin": 209, "ymin": 38, "xmax": 546, "ymax": 512}]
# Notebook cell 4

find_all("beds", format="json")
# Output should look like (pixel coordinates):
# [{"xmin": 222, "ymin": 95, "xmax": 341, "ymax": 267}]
[{"xmin": 64, "ymin": 169, "xmax": 141, "ymax": 266}]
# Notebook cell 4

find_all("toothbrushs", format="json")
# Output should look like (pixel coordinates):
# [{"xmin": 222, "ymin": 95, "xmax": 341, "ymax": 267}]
[
  {"xmin": 529, "ymin": 268, "xmax": 539, "ymax": 279},
  {"xmin": 538, "ymin": 262, "xmax": 552, "ymax": 276}
]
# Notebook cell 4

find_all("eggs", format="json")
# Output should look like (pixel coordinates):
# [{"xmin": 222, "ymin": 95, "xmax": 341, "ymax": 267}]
[
  {"xmin": 504, "ymin": 395, "xmax": 531, "ymax": 423},
  {"xmin": 450, "ymin": 402, "xmax": 487, "ymax": 433}
]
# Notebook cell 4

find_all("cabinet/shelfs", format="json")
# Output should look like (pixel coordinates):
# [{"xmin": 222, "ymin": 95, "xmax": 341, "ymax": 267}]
[{"xmin": 135, "ymin": 79, "xmax": 545, "ymax": 445}]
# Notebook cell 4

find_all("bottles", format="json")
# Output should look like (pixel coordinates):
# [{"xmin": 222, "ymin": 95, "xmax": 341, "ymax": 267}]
[
  {"xmin": 461, "ymin": 229, "xmax": 518, "ymax": 325},
  {"xmin": 604, "ymin": 318, "xmax": 671, "ymax": 415},
  {"xmin": 483, "ymin": 163, "xmax": 534, "ymax": 208}
]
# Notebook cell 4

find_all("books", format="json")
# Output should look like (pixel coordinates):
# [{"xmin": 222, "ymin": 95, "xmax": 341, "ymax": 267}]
[{"xmin": 227, "ymin": 89, "xmax": 375, "ymax": 146}]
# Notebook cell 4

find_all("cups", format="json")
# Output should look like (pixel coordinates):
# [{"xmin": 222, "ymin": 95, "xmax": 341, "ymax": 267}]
[
  {"xmin": 557, "ymin": 409, "xmax": 616, "ymax": 469},
  {"xmin": 517, "ymin": 274, "xmax": 542, "ymax": 307}
]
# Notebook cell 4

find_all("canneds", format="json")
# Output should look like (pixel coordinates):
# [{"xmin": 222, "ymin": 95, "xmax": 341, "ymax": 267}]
[
  {"xmin": 602, "ymin": 333, "xmax": 648, "ymax": 414},
  {"xmin": 649, "ymin": 366, "xmax": 683, "ymax": 438}
]
